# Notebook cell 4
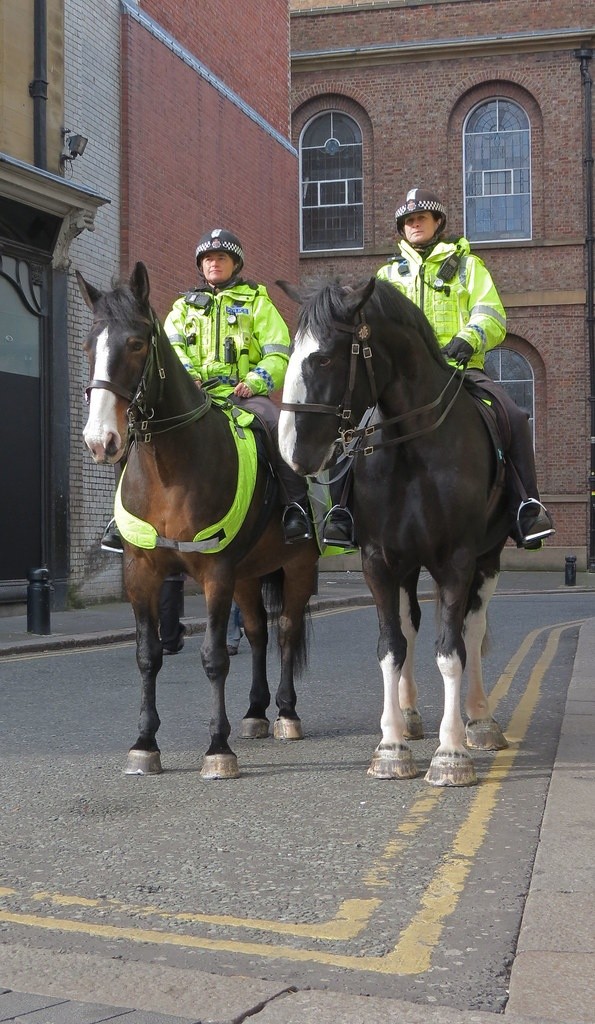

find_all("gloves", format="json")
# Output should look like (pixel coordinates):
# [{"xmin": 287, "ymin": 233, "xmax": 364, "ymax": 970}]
[{"xmin": 441, "ymin": 334, "xmax": 476, "ymax": 366}]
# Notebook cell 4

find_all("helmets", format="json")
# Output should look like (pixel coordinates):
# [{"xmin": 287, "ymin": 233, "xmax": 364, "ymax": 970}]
[
  {"xmin": 194, "ymin": 229, "xmax": 246, "ymax": 276},
  {"xmin": 395, "ymin": 189, "xmax": 447, "ymax": 236}
]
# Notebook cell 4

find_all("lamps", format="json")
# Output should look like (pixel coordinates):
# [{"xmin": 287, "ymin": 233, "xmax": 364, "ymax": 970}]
[{"xmin": 61, "ymin": 135, "xmax": 87, "ymax": 160}]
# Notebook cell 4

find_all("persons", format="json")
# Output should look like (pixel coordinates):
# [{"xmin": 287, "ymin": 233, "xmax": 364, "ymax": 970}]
[
  {"xmin": 102, "ymin": 229, "xmax": 316, "ymax": 549},
  {"xmin": 322, "ymin": 189, "xmax": 549, "ymax": 551}
]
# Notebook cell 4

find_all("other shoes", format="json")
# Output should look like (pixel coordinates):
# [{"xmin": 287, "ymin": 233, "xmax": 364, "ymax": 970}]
[
  {"xmin": 325, "ymin": 522, "xmax": 352, "ymax": 549},
  {"xmin": 226, "ymin": 644, "xmax": 238, "ymax": 655},
  {"xmin": 162, "ymin": 625, "xmax": 186, "ymax": 654},
  {"xmin": 286, "ymin": 520, "xmax": 308, "ymax": 537},
  {"xmin": 101, "ymin": 528, "xmax": 124, "ymax": 553},
  {"xmin": 514, "ymin": 514, "xmax": 553, "ymax": 547}
]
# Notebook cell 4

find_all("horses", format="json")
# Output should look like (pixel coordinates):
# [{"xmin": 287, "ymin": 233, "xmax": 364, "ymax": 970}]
[
  {"xmin": 75, "ymin": 259, "xmax": 319, "ymax": 780},
  {"xmin": 274, "ymin": 276, "xmax": 510, "ymax": 785}
]
